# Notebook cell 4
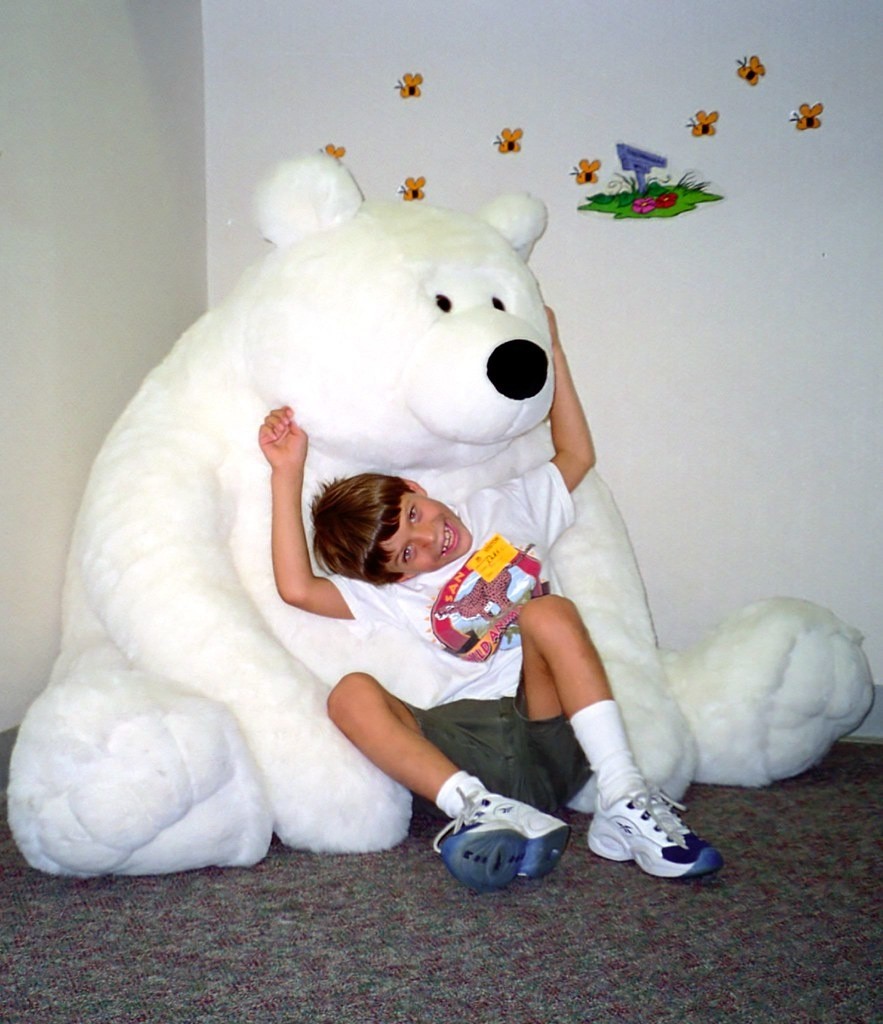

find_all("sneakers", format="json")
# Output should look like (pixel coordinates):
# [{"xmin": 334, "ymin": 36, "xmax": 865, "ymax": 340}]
[
  {"xmin": 432, "ymin": 787, "xmax": 572, "ymax": 893},
  {"xmin": 588, "ymin": 791, "xmax": 724, "ymax": 877}
]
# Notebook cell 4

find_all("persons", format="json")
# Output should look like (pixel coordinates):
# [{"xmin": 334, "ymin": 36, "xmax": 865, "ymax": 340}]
[{"xmin": 256, "ymin": 306, "xmax": 725, "ymax": 890}]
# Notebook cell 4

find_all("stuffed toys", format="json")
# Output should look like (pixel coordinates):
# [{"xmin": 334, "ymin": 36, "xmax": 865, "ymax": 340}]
[{"xmin": 5, "ymin": 148, "xmax": 872, "ymax": 876}]
